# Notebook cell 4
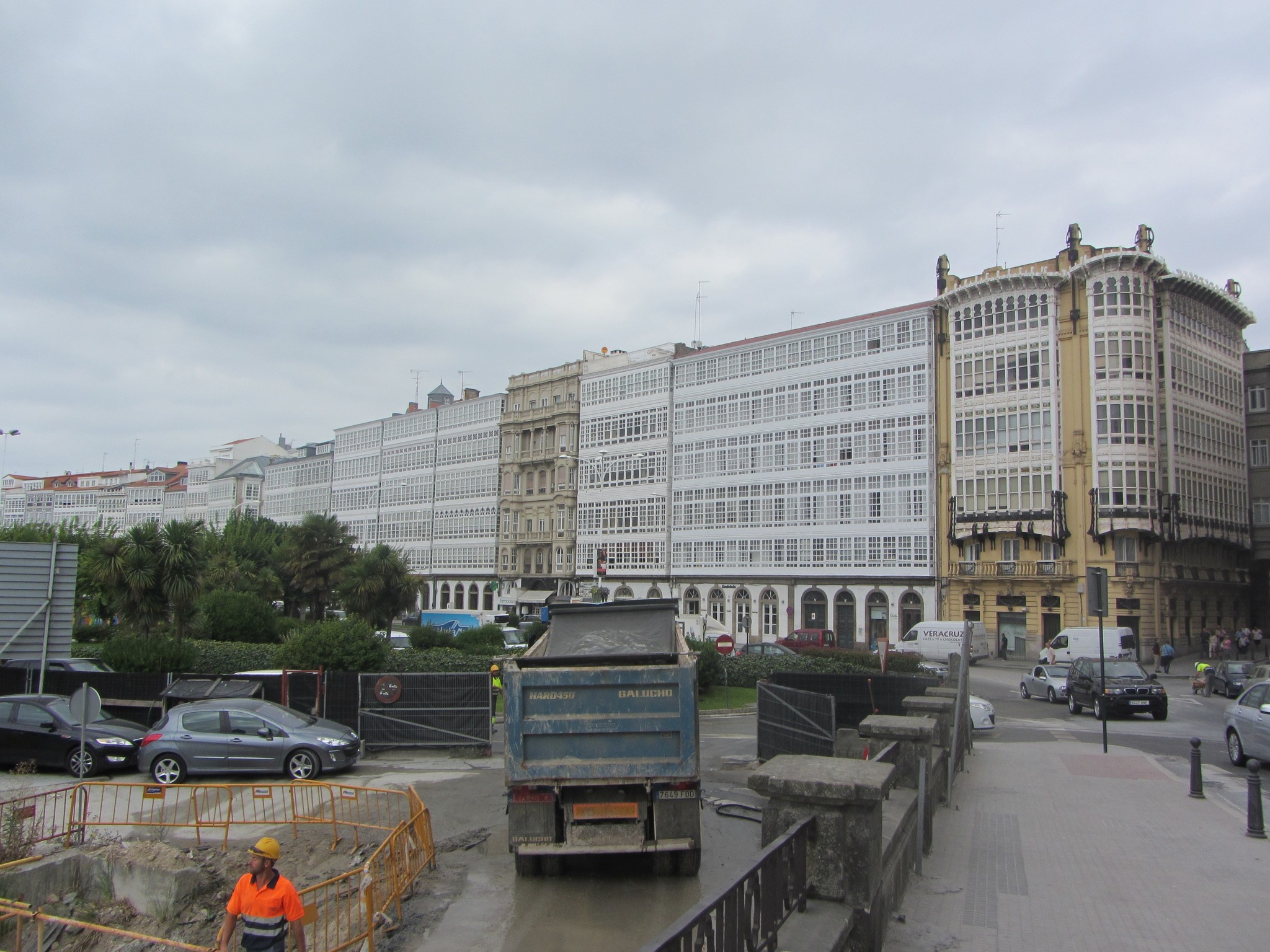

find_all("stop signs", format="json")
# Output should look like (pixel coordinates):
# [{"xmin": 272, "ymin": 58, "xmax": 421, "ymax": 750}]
[{"xmin": 716, "ymin": 634, "xmax": 734, "ymax": 654}]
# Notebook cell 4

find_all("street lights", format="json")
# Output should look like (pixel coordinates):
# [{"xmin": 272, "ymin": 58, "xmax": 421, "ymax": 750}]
[{"xmin": 364, "ymin": 483, "xmax": 408, "ymax": 552}]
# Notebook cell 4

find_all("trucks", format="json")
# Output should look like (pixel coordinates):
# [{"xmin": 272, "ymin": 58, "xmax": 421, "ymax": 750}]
[{"xmin": 493, "ymin": 595, "xmax": 706, "ymax": 878}]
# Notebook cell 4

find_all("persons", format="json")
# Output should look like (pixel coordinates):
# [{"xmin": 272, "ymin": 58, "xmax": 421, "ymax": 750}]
[
  {"xmin": 1219, "ymin": 635, "xmax": 1231, "ymax": 660},
  {"xmin": 600, "ymin": 549, "xmax": 606, "ymax": 564},
  {"xmin": 1252, "ymin": 627, "xmax": 1262, "ymax": 647},
  {"xmin": 1045, "ymin": 642, "xmax": 1056, "ymax": 665},
  {"xmin": 1162, "ymin": 641, "xmax": 1174, "ymax": 673},
  {"xmin": 597, "ymin": 559, "xmax": 602, "ymax": 569},
  {"xmin": 1238, "ymin": 633, "xmax": 1250, "ymax": 652},
  {"xmin": 1193, "ymin": 662, "xmax": 1215, "ymax": 698},
  {"xmin": 490, "ymin": 665, "xmax": 504, "ymax": 732},
  {"xmin": 1001, "ymin": 633, "xmax": 1008, "ymax": 661},
  {"xmin": 1215, "ymin": 625, "xmax": 1225, "ymax": 637},
  {"xmin": 220, "ymin": 837, "xmax": 306, "ymax": 952},
  {"xmin": 1241, "ymin": 626, "xmax": 1251, "ymax": 639},
  {"xmin": 1236, "ymin": 628, "xmax": 1243, "ymax": 648},
  {"xmin": 1208, "ymin": 631, "xmax": 1217, "ymax": 660},
  {"xmin": 1201, "ymin": 628, "xmax": 1210, "ymax": 657},
  {"xmin": 1152, "ymin": 638, "xmax": 1161, "ymax": 673}
]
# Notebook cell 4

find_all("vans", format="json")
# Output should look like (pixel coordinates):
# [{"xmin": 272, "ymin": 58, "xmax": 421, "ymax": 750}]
[
  {"xmin": 893, "ymin": 621, "xmax": 993, "ymax": 665},
  {"xmin": 1036, "ymin": 627, "xmax": 1138, "ymax": 665},
  {"xmin": 771, "ymin": 629, "xmax": 836, "ymax": 651}
]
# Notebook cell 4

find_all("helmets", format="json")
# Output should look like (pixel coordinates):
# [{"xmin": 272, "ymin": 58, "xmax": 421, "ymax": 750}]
[
  {"xmin": 248, "ymin": 837, "xmax": 281, "ymax": 859},
  {"xmin": 490, "ymin": 665, "xmax": 499, "ymax": 671},
  {"xmin": 1194, "ymin": 662, "xmax": 1199, "ymax": 667}
]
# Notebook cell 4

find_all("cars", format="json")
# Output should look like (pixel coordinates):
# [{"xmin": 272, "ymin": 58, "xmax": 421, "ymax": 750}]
[
  {"xmin": 734, "ymin": 642, "xmax": 799, "ymax": 658},
  {"xmin": 968, "ymin": 695, "xmax": 997, "ymax": 730},
  {"xmin": 873, "ymin": 650, "xmax": 949, "ymax": 681},
  {"xmin": 1223, "ymin": 681, "xmax": 1270, "ymax": 768},
  {"xmin": 401, "ymin": 612, "xmax": 420, "ymax": 626},
  {"xmin": 136, "ymin": 696, "xmax": 361, "ymax": 788},
  {"xmin": 1211, "ymin": 660, "xmax": 1262, "ymax": 698},
  {"xmin": 1239, "ymin": 666, "xmax": 1270, "ymax": 692},
  {"xmin": 0, "ymin": 694, "xmax": 152, "ymax": 779},
  {"xmin": 324, "ymin": 609, "xmax": 347, "ymax": 622},
  {"xmin": 1019, "ymin": 664, "xmax": 1071, "ymax": 705},
  {"xmin": 4, "ymin": 658, "xmax": 115, "ymax": 673},
  {"xmin": 373, "ymin": 630, "xmax": 412, "ymax": 650},
  {"xmin": 273, "ymin": 601, "xmax": 284, "ymax": 611}
]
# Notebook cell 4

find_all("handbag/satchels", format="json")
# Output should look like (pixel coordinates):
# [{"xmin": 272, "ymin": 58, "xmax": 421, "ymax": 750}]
[{"xmin": 1169, "ymin": 656, "xmax": 1174, "ymax": 661}]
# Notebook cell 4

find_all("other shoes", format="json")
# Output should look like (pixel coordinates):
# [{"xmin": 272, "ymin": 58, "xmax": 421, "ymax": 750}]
[
  {"xmin": 1155, "ymin": 670, "xmax": 1161, "ymax": 673},
  {"xmin": 492, "ymin": 727, "xmax": 497, "ymax": 732}
]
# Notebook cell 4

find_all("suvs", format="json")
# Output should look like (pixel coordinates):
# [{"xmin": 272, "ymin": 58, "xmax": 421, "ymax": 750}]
[{"xmin": 1064, "ymin": 657, "xmax": 1168, "ymax": 720}]
